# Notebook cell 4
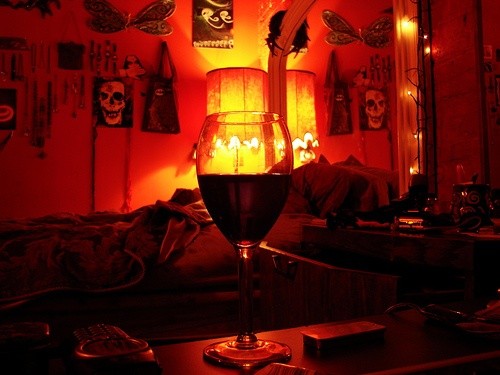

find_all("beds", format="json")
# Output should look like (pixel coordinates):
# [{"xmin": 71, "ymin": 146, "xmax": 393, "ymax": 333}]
[{"xmin": 5, "ymin": 165, "xmax": 374, "ymax": 335}]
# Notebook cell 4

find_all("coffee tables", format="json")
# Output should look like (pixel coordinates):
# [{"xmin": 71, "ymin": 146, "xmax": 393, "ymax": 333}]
[{"xmin": 146, "ymin": 302, "xmax": 500, "ymax": 375}]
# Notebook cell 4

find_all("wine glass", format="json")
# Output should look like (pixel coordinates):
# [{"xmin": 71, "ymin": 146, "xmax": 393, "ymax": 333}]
[{"xmin": 195, "ymin": 110, "xmax": 294, "ymax": 370}]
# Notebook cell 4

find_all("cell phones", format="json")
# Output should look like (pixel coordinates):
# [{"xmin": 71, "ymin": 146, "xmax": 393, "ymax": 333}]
[{"xmin": 63, "ymin": 321, "xmax": 162, "ymax": 375}]
